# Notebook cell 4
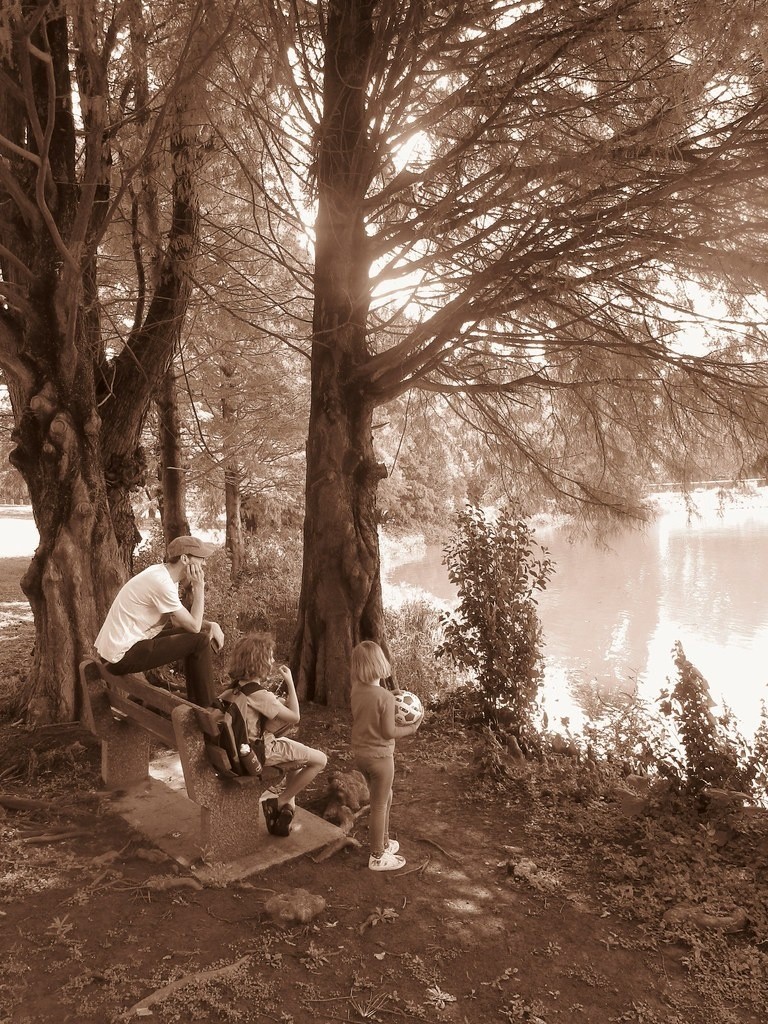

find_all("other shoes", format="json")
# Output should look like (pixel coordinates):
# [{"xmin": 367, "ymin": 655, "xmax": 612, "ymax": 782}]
[
  {"xmin": 262, "ymin": 797, "xmax": 279, "ymax": 834},
  {"xmin": 277, "ymin": 803, "xmax": 294, "ymax": 836}
]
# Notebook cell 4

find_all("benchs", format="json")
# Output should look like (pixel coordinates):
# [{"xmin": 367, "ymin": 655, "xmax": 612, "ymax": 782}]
[{"xmin": 79, "ymin": 660, "xmax": 302, "ymax": 864}]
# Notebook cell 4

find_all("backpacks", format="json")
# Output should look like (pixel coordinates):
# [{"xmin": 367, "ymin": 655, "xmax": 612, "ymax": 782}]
[{"xmin": 204, "ymin": 680, "xmax": 267, "ymax": 775}]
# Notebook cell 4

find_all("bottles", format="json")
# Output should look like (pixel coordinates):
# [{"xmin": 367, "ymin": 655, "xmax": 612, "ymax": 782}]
[{"xmin": 241, "ymin": 742, "xmax": 262, "ymax": 776}]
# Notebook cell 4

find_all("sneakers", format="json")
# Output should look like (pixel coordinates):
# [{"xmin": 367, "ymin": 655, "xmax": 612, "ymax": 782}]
[
  {"xmin": 384, "ymin": 839, "xmax": 399, "ymax": 855},
  {"xmin": 369, "ymin": 851, "xmax": 406, "ymax": 871}
]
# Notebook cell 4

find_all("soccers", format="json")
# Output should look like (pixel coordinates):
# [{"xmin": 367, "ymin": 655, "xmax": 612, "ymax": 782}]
[{"xmin": 393, "ymin": 690, "xmax": 423, "ymax": 725}]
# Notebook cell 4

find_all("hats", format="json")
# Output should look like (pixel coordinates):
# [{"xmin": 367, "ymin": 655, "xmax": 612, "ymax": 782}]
[{"xmin": 167, "ymin": 536, "xmax": 214, "ymax": 557}]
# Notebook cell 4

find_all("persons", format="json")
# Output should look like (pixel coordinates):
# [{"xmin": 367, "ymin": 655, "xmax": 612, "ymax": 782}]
[
  {"xmin": 93, "ymin": 536, "xmax": 225, "ymax": 708},
  {"xmin": 213, "ymin": 632, "xmax": 327, "ymax": 837},
  {"xmin": 349, "ymin": 640, "xmax": 426, "ymax": 871}
]
